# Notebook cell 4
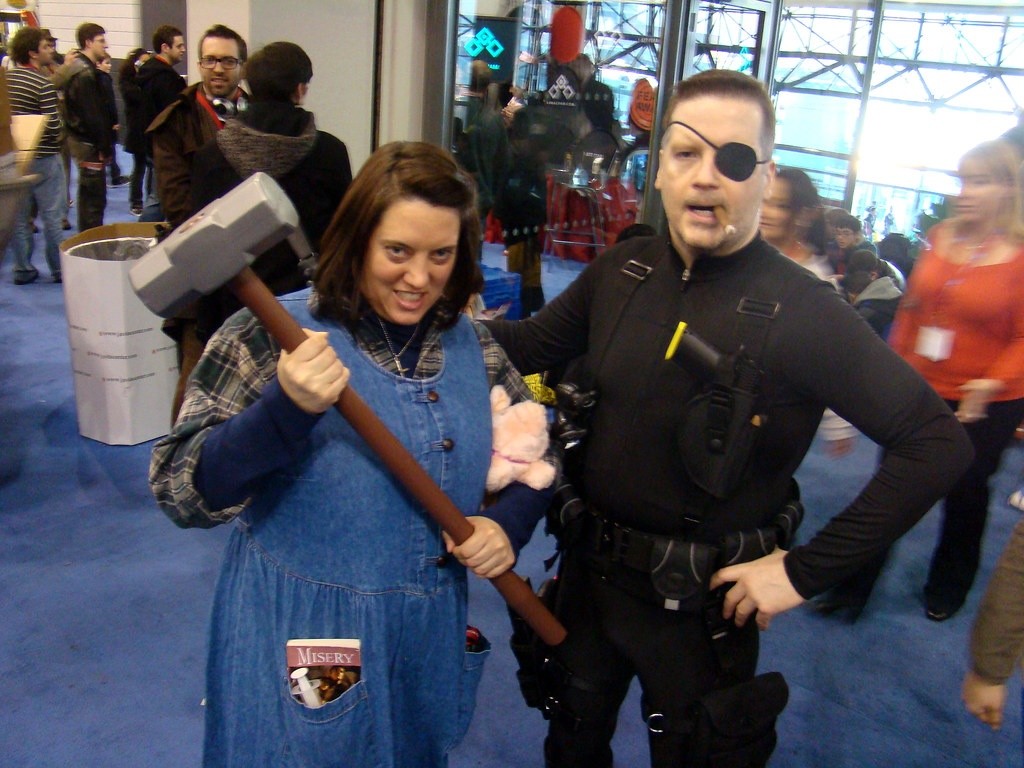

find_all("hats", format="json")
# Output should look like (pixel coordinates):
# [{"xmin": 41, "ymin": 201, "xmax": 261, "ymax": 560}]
[{"xmin": 40, "ymin": 28, "xmax": 58, "ymax": 39}]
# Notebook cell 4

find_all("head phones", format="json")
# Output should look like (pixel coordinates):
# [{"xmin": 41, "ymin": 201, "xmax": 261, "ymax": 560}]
[{"xmin": 206, "ymin": 90, "xmax": 248, "ymax": 116}]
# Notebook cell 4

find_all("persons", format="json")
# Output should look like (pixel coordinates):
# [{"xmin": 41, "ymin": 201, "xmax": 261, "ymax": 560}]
[
  {"xmin": 7, "ymin": 25, "xmax": 188, "ymax": 284},
  {"xmin": 317, "ymin": 666, "xmax": 347, "ymax": 704},
  {"xmin": 756, "ymin": 109, "xmax": 1024, "ymax": 729},
  {"xmin": 454, "ymin": 5, "xmax": 656, "ymax": 317},
  {"xmin": 145, "ymin": 24, "xmax": 352, "ymax": 425},
  {"xmin": 146, "ymin": 141, "xmax": 560, "ymax": 768},
  {"xmin": 477, "ymin": 69, "xmax": 972, "ymax": 768}
]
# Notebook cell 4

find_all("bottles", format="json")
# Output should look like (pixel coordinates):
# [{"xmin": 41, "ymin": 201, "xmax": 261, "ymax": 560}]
[{"xmin": 480, "ymin": 208, "xmax": 507, "ymax": 273}]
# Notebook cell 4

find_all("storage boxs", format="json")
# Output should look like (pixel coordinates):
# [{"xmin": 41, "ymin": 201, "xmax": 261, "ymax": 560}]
[{"xmin": 60, "ymin": 220, "xmax": 179, "ymax": 446}]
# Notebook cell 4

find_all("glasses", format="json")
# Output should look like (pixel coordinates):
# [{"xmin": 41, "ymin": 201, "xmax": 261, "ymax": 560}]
[
  {"xmin": 833, "ymin": 230, "xmax": 855, "ymax": 237},
  {"xmin": 199, "ymin": 55, "xmax": 242, "ymax": 71}
]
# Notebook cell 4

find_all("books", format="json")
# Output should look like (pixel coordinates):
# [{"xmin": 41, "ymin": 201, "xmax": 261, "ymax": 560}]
[{"xmin": 286, "ymin": 638, "xmax": 362, "ymax": 707}]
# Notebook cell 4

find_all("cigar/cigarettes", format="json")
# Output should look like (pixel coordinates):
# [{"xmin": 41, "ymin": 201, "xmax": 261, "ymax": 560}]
[{"xmin": 715, "ymin": 206, "xmax": 736, "ymax": 237}]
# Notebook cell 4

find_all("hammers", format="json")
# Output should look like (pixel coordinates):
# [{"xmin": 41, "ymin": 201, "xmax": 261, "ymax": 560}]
[{"xmin": 128, "ymin": 172, "xmax": 569, "ymax": 654}]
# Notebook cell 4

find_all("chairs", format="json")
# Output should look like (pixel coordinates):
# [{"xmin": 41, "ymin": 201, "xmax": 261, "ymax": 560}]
[{"xmin": 542, "ymin": 130, "xmax": 618, "ymax": 273}]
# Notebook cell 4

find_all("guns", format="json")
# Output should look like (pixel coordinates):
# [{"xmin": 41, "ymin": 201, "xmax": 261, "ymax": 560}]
[
  {"xmin": 507, "ymin": 574, "xmax": 557, "ymax": 644},
  {"xmin": 663, "ymin": 321, "xmax": 766, "ymax": 390}
]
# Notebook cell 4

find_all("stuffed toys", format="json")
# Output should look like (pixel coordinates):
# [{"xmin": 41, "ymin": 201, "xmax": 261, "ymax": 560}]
[{"xmin": 487, "ymin": 383, "xmax": 556, "ymax": 493}]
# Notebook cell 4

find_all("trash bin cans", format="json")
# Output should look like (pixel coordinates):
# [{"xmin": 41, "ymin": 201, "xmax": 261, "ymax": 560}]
[{"xmin": 59, "ymin": 218, "xmax": 179, "ymax": 445}]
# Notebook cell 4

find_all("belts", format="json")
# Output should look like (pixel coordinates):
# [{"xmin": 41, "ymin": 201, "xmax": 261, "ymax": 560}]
[{"xmin": 544, "ymin": 481, "xmax": 805, "ymax": 597}]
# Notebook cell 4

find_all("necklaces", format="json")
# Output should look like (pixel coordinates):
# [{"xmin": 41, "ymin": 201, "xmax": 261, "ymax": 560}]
[
  {"xmin": 934, "ymin": 222, "xmax": 998, "ymax": 302},
  {"xmin": 374, "ymin": 313, "xmax": 419, "ymax": 379}
]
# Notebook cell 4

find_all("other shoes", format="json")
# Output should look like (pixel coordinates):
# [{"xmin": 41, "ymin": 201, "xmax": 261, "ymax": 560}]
[
  {"xmin": 925, "ymin": 592, "xmax": 967, "ymax": 621},
  {"xmin": 112, "ymin": 176, "xmax": 131, "ymax": 187},
  {"xmin": 129, "ymin": 205, "xmax": 144, "ymax": 216},
  {"xmin": 50, "ymin": 271, "xmax": 62, "ymax": 283},
  {"xmin": 33, "ymin": 226, "xmax": 39, "ymax": 233},
  {"xmin": 61, "ymin": 220, "xmax": 72, "ymax": 230},
  {"xmin": 69, "ymin": 200, "xmax": 75, "ymax": 206},
  {"xmin": 14, "ymin": 269, "xmax": 38, "ymax": 284},
  {"xmin": 813, "ymin": 593, "xmax": 864, "ymax": 623}
]
